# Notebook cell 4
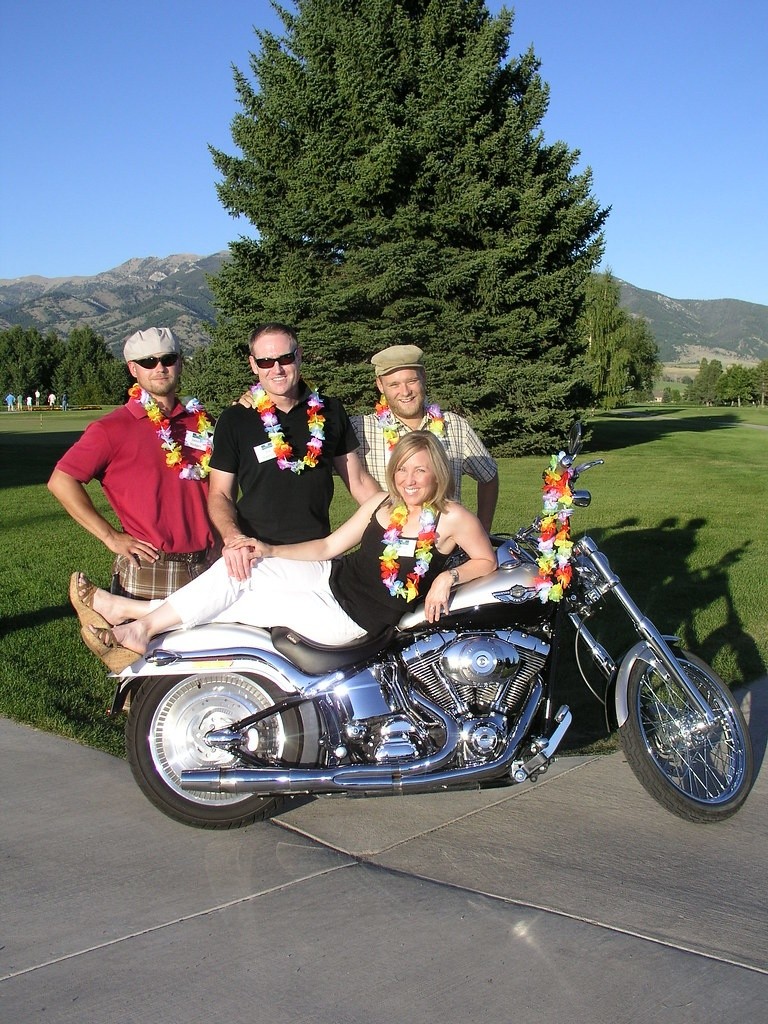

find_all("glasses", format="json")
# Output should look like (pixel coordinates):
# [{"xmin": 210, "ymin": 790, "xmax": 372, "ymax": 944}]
[
  {"xmin": 251, "ymin": 348, "xmax": 297, "ymax": 368},
  {"xmin": 133, "ymin": 354, "xmax": 178, "ymax": 369}
]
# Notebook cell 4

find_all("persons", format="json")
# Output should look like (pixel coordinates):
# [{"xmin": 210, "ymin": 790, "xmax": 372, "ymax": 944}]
[
  {"xmin": 70, "ymin": 430, "xmax": 497, "ymax": 676},
  {"xmin": 208, "ymin": 323, "xmax": 384, "ymax": 581},
  {"xmin": 6, "ymin": 390, "xmax": 69, "ymax": 411},
  {"xmin": 46, "ymin": 328, "xmax": 224, "ymax": 710},
  {"xmin": 231, "ymin": 344, "xmax": 499, "ymax": 533}
]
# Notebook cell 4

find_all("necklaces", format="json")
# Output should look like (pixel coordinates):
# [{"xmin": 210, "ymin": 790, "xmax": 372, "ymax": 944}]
[
  {"xmin": 128, "ymin": 383, "xmax": 215, "ymax": 480},
  {"xmin": 374, "ymin": 394, "xmax": 446, "ymax": 450},
  {"xmin": 534, "ymin": 451, "xmax": 574, "ymax": 603},
  {"xmin": 251, "ymin": 383, "xmax": 325, "ymax": 475},
  {"xmin": 379, "ymin": 501, "xmax": 437, "ymax": 603}
]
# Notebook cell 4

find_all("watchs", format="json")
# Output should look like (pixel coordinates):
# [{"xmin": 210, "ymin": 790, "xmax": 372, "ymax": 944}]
[{"xmin": 446, "ymin": 569, "xmax": 459, "ymax": 583}]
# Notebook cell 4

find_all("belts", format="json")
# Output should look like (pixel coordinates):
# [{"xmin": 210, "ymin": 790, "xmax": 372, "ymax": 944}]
[{"xmin": 164, "ymin": 549, "xmax": 206, "ymax": 565}]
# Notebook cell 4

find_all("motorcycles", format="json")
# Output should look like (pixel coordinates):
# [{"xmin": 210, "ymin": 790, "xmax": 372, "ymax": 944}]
[{"xmin": 104, "ymin": 421, "xmax": 754, "ymax": 830}]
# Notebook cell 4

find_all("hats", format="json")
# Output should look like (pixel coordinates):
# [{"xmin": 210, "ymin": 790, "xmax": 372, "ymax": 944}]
[
  {"xmin": 371, "ymin": 345, "xmax": 424, "ymax": 377},
  {"xmin": 124, "ymin": 327, "xmax": 180, "ymax": 362}
]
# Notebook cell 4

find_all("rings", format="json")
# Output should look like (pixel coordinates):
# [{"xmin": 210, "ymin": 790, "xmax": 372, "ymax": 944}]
[{"xmin": 429, "ymin": 606, "xmax": 435, "ymax": 609}]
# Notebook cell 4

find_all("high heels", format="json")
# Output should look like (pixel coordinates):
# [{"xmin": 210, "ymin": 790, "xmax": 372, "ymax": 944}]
[
  {"xmin": 69, "ymin": 572, "xmax": 112, "ymax": 629},
  {"xmin": 81, "ymin": 623, "xmax": 143, "ymax": 675}
]
